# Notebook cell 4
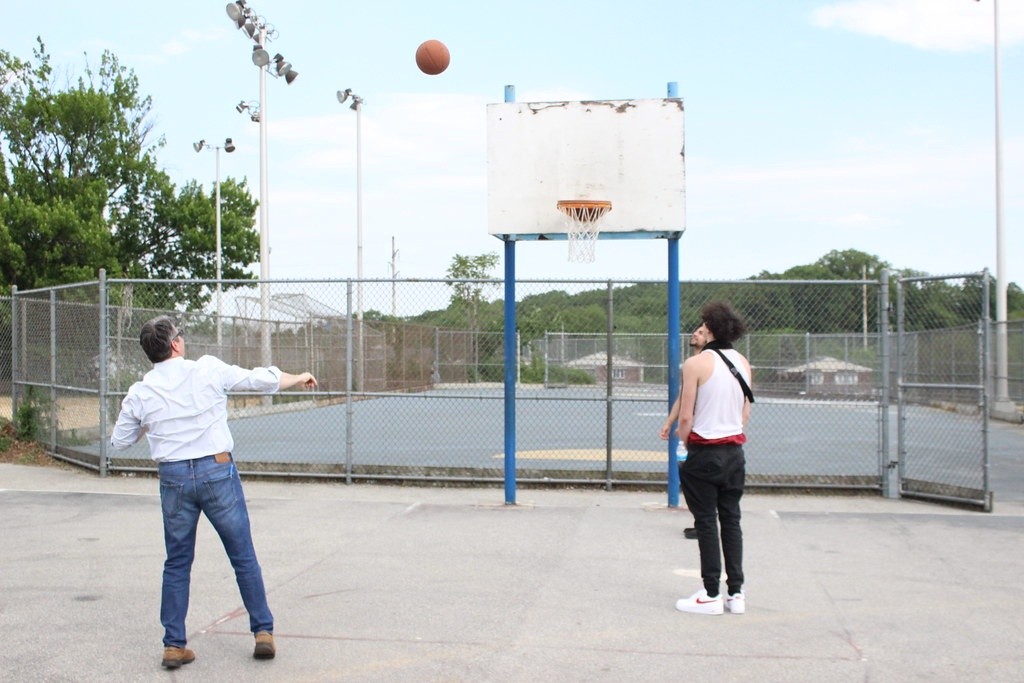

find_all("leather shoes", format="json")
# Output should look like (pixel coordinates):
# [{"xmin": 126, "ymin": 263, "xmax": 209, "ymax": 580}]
[
  {"xmin": 254, "ymin": 631, "xmax": 275, "ymax": 658},
  {"xmin": 161, "ymin": 647, "xmax": 195, "ymax": 668}
]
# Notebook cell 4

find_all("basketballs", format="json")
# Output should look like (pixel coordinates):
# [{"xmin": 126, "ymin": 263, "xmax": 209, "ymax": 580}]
[{"xmin": 415, "ymin": 39, "xmax": 451, "ymax": 75}]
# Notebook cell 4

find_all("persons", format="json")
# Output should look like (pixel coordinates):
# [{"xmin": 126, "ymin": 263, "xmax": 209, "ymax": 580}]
[
  {"xmin": 661, "ymin": 301, "xmax": 752, "ymax": 614},
  {"xmin": 110, "ymin": 316, "xmax": 318, "ymax": 668}
]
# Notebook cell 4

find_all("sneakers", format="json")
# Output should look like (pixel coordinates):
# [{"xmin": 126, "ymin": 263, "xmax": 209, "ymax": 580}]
[
  {"xmin": 725, "ymin": 590, "xmax": 746, "ymax": 614},
  {"xmin": 676, "ymin": 589, "xmax": 724, "ymax": 614}
]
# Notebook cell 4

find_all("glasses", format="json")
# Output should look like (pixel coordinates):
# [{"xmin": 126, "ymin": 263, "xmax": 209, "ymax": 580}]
[{"xmin": 171, "ymin": 329, "xmax": 184, "ymax": 347}]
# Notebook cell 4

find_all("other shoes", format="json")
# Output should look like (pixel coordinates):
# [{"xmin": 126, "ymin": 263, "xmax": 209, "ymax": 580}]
[{"xmin": 684, "ymin": 528, "xmax": 698, "ymax": 539}]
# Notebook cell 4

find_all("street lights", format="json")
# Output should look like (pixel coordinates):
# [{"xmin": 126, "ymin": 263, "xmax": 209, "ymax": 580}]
[
  {"xmin": 225, "ymin": 0, "xmax": 298, "ymax": 405},
  {"xmin": 193, "ymin": 138, "xmax": 236, "ymax": 359},
  {"xmin": 337, "ymin": 88, "xmax": 362, "ymax": 398}
]
